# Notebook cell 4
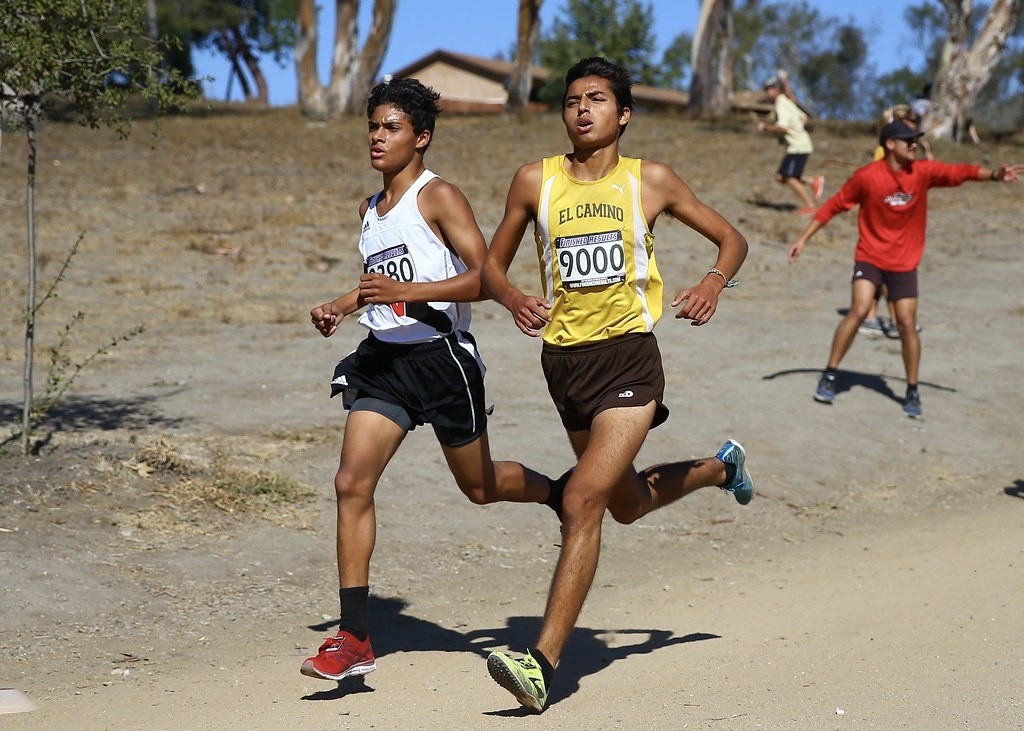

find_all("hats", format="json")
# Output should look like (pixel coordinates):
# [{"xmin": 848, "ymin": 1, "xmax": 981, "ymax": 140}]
[{"xmin": 880, "ymin": 122, "xmax": 925, "ymax": 146}]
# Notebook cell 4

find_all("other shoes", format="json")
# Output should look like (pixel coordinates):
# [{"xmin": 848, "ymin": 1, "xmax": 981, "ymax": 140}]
[
  {"xmin": 811, "ymin": 176, "xmax": 824, "ymax": 200},
  {"xmin": 791, "ymin": 207, "xmax": 817, "ymax": 216}
]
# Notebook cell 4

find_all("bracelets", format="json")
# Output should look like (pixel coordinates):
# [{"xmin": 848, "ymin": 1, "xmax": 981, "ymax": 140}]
[
  {"xmin": 705, "ymin": 268, "xmax": 741, "ymax": 289},
  {"xmin": 989, "ymin": 166, "xmax": 1000, "ymax": 183}
]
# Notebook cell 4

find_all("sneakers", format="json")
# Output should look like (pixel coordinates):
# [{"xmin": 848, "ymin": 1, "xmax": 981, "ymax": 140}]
[
  {"xmin": 487, "ymin": 648, "xmax": 560, "ymax": 713},
  {"xmin": 717, "ymin": 438, "xmax": 755, "ymax": 505},
  {"xmin": 857, "ymin": 317, "xmax": 889, "ymax": 336},
  {"xmin": 904, "ymin": 390, "xmax": 922, "ymax": 416},
  {"xmin": 300, "ymin": 631, "xmax": 377, "ymax": 681},
  {"xmin": 814, "ymin": 372, "xmax": 837, "ymax": 403},
  {"xmin": 888, "ymin": 321, "xmax": 921, "ymax": 338}
]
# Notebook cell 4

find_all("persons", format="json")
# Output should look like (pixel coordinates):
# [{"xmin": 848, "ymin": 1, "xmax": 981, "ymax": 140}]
[
  {"xmin": 785, "ymin": 118, "xmax": 1024, "ymax": 417},
  {"xmin": 747, "ymin": 76, "xmax": 846, "ymax": 216},
  {"xmin": 907, "ymin": 82, "xmax": 983, "ymax": 144},
  {"xmin": 853, "ymin": 104, "xmax": 936, "ymax": 341},
  {"xmin": 299, "ymin": 77, "xmax": 576, "ymax": 681},
  {"xmin": 474, "ymin": 56, "xmax": 756, "ymax": 714}
]
